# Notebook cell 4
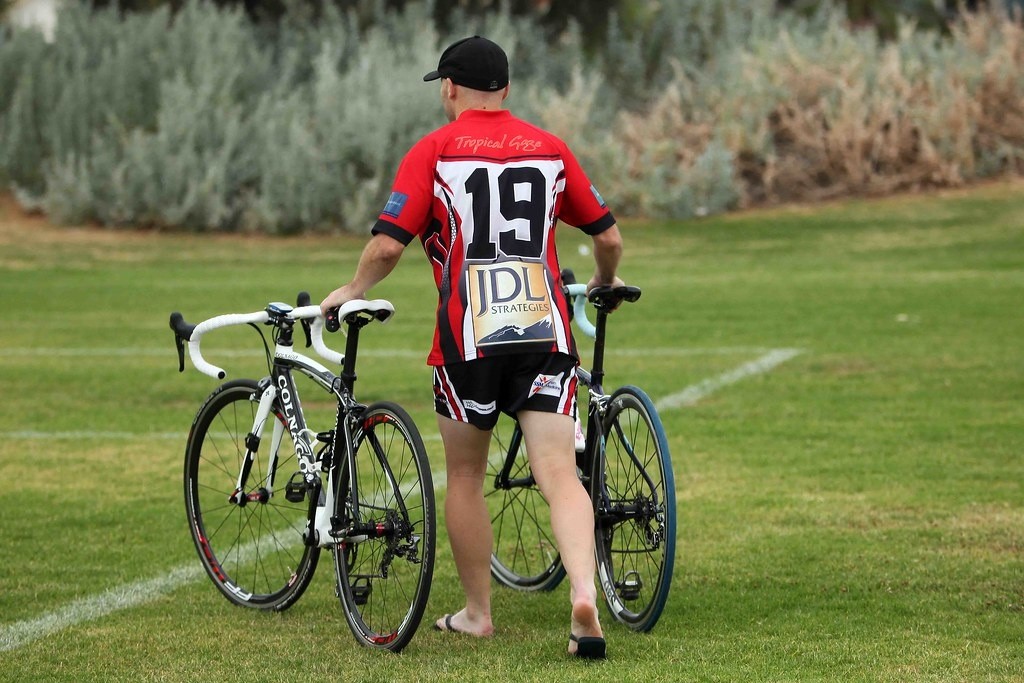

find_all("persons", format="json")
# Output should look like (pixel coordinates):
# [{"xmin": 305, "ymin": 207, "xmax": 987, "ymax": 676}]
[{"xmin": 320, "ymin": 34, "xmax": 624, "ymax": 657}]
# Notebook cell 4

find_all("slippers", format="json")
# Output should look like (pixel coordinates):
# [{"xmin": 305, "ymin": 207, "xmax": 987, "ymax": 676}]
[
  {"xmin": 569, "ymin": 633, "xmax": 606, "ymax": 662},
  {"xmin": 433, "ymin": 616, "xmax": 457, "ymax": 632}
]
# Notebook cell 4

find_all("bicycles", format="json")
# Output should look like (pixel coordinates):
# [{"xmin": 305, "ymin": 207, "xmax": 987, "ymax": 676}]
[
  {"xmin": 484, "ymin": 269, "xmax": 677, "ymax": 632},
  {"xmin": 168, "ymin": 292, "xmax": 438, "ymax": 653}
]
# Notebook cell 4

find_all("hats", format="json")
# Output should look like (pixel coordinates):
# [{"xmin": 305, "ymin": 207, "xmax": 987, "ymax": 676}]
[{"xmin": 423, "ymin": 34, "xmax": 509, "ymax": 92}]
